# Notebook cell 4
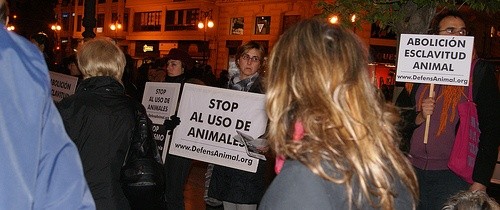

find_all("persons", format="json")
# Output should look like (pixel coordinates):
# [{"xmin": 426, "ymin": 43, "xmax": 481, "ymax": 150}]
[
  {"xmin": 55, "ymin": 36, "xmax": 146, "ymax": 210},
  {"xmin": 394, "ymin": 9, "xmax": 500, "ymax": 210},
  {"xmin": 32, "ymin": 31, "xmax": 229, "ymax": 103},
  {"xmin": 202, "ymin": 39, "xmax": 277, "ymax": 210},
  {"xmin": 0, "ymin": 0, "xmax": 96, "ymax": 210},
  {"xmin": 257, "ymin": 20, "xmax": 419, "ymax": 210},
  {"xmin": 441, "ymin": 190, "xmax": 500, "ymax": 210}
]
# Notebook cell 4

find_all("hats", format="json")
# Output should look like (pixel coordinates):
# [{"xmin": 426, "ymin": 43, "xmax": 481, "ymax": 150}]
[{"xmin": 167, "ymin": 48, "xmax": 193, "ymax": 70}]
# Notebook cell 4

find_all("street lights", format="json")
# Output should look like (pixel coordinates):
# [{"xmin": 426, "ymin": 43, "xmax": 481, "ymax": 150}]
[
  {"xmin": 110, "ymin": 19, "xmax": 122, "ymax": 45},
  {"xmin": 197, "ymin": 16, "xmax": 214, "ymax": 65}
]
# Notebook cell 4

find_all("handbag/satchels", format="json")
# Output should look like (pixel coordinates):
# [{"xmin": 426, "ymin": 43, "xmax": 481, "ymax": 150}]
[
  {"xmin": 448, "ymin": 58, "xmax": 482, "ymax": 185},
  {"xmin": 203, "ymin": 164, "xmax": 222, "ymax": 206},
  {"xmin": 119, "ymin": 104, "xmax": 167, "ymax": 210}
]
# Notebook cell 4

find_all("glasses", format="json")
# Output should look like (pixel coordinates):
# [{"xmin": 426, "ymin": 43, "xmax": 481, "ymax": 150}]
[
  {"xmin": 242, "ymin": 54, "xmax": 260, "ymax": 62},
  {"xmin": 442, "ymin": 27, "xmax": 470, "ymax": 34}
]
